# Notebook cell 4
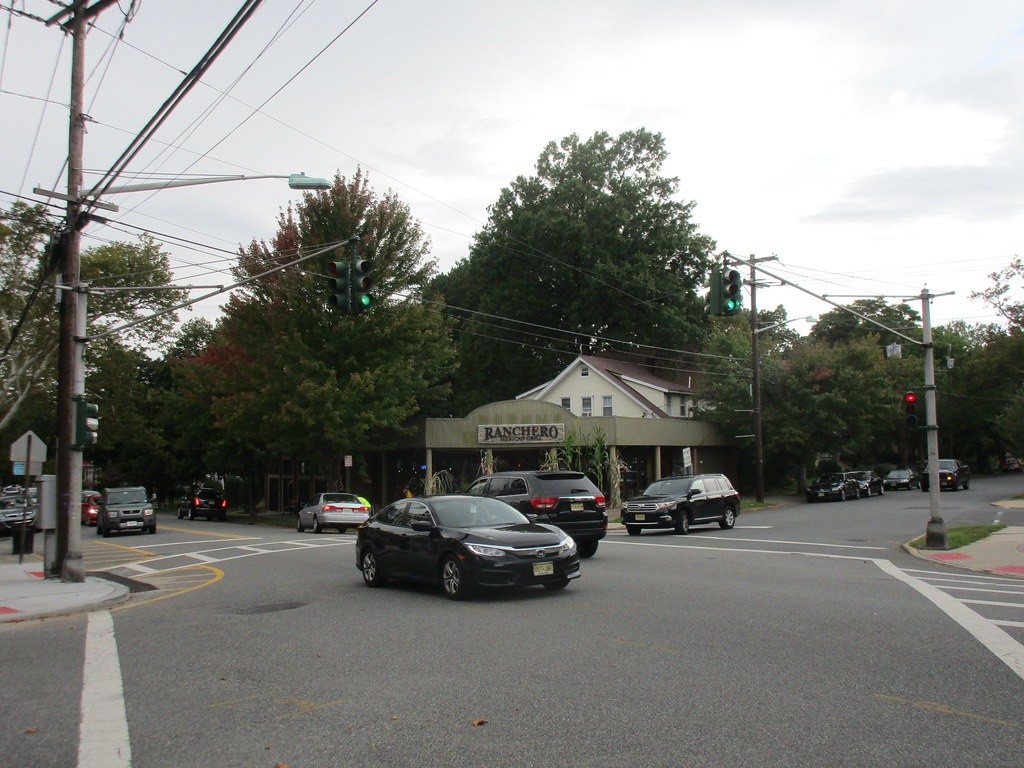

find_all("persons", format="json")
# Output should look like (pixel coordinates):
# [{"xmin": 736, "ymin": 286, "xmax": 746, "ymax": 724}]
[
  {"xmin": 354, "ymin": 493, "xmax": 372, "ymax": 517},
  {"xmin": 402, "ymin": 485, "xmax": 414, "ymax": 498}
]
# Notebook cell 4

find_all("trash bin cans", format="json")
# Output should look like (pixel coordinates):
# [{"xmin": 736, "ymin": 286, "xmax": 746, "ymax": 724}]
[{"xmin": 12, "ymin": 527, "xmax": 35, "ymax": 554}]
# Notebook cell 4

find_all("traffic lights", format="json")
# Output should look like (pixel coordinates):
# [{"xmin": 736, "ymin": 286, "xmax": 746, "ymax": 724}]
[
  {"xmin": 327, "ymin": 260, "xmax": 348, "ymax": 313},
  {"xmin": 350, "ymin": 258, "xmax": 374, "ymax": 313},
  {"xmin": 905, "ymin": 391, "xmax": 918, "ymax": 427},
  {"xmin": 718, "ymin": 268, "xmax": 742, "ymax": 316}
]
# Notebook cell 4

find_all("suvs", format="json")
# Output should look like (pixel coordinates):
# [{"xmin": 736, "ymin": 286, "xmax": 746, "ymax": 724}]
[
  {"xmin": 620, "ymin": 471, "xmax": 746, "ymax": 534},
  {"xmin": 453, "ymin": 470, "xmax": 608, "ymax": 559}
]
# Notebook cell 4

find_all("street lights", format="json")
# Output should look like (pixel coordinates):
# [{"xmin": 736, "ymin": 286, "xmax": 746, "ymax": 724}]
[{"xmin": 46, "ymin": 167, "xmax": 336, "ymax": 585}]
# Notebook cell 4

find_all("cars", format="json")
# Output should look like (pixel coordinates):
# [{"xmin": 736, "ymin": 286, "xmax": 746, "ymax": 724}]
[
  {"xmin": 807, "ymin": 472, "xmax": 862, "ymax": 501},
  {"xmin": 22, "ymin": 487, "xmax": 37, "ymax": 510},
  {"xmin": 96, "ymin": 485, "xmax": 158, "ymax": 536},
  {"xmin": 177, "ymin": 489, "xmax": 227, "ymax": 521},
  {"xmin": 881, "ymin": 467, "xmax": 916, "ymax": 492},
  {"xmin": 294, "ymin": 492, "xmax": 371, "ymax": 536},
  {"xmin": 80, "ymin": 490, "xmax": 101, "ymax": 525},
  {"xmin": 352, "ymin": 494, "xmax": 583, "ymax": 601},
  {"xmin": 846, "ymin": 469, "xmax": 883, "ymax": 498},
  {"xmin": 0, "ymin": 492, "xmax": 37, "ymax": 532},
  {"xmin": 1002, "ymin": 458, "xmax": 1021, "ymax": 472},
  {"xmin": 918, "ymin": 458, "xmax": 970, "ymax": 492}
]
